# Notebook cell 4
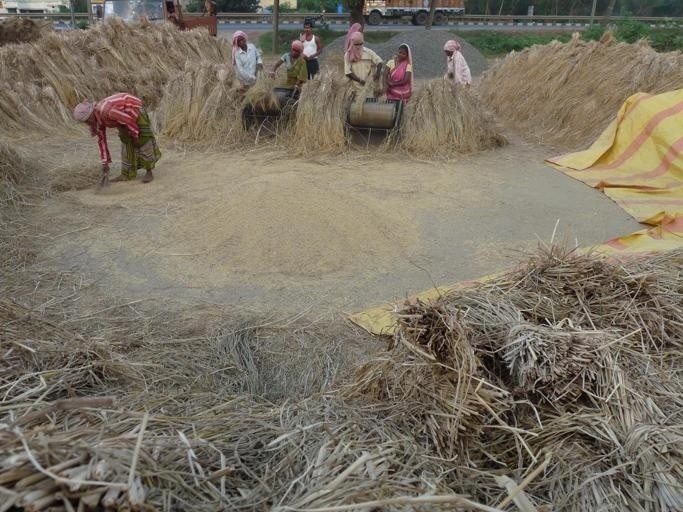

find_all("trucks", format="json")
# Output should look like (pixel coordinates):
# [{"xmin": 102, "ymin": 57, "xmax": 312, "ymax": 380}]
[
  {"xmin": 362, "ymin": 1, "xmax": 465, "ymax": 26},
  {"xmin": 96, "ymin": 0, "xmax": 218, "ymax": 38}
]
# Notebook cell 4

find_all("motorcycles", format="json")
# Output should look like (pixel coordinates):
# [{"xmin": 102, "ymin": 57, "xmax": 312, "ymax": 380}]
[{"xmin": 303, "ymin": 10, "xmax": 330, "ymax": 31}]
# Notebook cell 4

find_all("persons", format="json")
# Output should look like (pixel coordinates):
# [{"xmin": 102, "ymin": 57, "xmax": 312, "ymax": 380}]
[
  {"xmin": 270, "ymin": 41, "xmax": 308, "ymax": 90},
  {"xmin": 299, "ymin": 20, "xmax": 323, "ymax": 80},
  {"xmin": 73, "ymin": 92, "xmax": 161, "ymax": 186},
  {"xmin": 384, "ymin": 43, "xmax": 413, "ymax": 105},
  {"xmin": 230, "ymin": 31, "xmax": 262, "ymax": 99},
  {"xmin": 344, "ymin": 23, "xmax": 361, "ymax": 52},
  {"xmin": 442, "ymin": 40, "xmax": 471, "ymax": 87},
  {"xmin": 342, "ymin": 32, "xmax": 383, "ymax": 99},
  {"xmin": 199, "ymin": 0, "xmax": 217, "ymax": 18}
]
